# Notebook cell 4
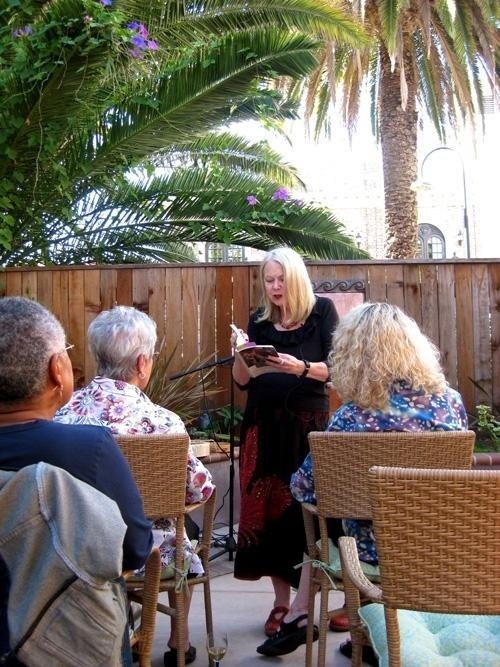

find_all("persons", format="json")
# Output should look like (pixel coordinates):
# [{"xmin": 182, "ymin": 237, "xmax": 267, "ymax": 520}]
[
  {"xmin": 233, "ymin": 247, "xmax": 354, "ymax": 637},
  {"xmin": 257, "ymin": 301, "xmax": 470, "ymax": 666},
  {"xmin": 51, "ymin": 304, "xmax": 217, "ymax": 667},
  {"xmin": 1, "ymin": 294, "xmax": 154, "ymax": 666}
]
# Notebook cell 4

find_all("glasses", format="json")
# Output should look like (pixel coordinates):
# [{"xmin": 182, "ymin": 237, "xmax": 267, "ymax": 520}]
[
  {"xmin": 48, "ymin": 340, "xmax": 75, "ymax": 366},
  {"xmin": 144, "ymin": 350, "xmax": 160, "ymax": 362}
]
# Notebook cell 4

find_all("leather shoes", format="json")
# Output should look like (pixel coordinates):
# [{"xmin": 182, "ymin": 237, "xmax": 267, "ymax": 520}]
[
  {"xmin": 265, "ymin": 606, "xmax": 289, "ymax": 637},
  {"xmin": 329, "ymin": 613, "xmax": 351, "ymax": 631}
]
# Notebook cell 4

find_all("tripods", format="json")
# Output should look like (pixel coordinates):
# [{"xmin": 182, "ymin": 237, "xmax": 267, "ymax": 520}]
[{"xmin": 209, "ymin": 376, "xmax": 253, "ymax": 560}]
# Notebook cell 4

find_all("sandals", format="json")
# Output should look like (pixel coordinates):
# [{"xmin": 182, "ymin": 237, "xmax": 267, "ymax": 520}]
[
  {"xmin": 339, "ymin": 639, "xmax": 379, "ymax": 666},
  {"xmin": 257, "ymin": 613, "xmax": 319, "ymax": 656}
]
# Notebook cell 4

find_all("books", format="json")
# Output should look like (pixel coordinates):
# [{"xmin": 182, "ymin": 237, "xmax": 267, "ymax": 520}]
[{"xmin": 229, "ymin": 323, "xmax": 282, "ymax": 378}]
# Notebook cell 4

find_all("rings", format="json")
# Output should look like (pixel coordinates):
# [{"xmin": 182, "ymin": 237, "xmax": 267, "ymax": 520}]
[{"xmin": 279, "ymin": 358, "xmax": 285, "ymax": 365}]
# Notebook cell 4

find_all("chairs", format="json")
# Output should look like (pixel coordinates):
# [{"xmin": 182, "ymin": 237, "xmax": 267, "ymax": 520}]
[
  {"xmin": 116, "ymin": 432, "xmax": 217, "ymax": 666},
  {"xmin": 294, "ymin": 427, "xmax": 476, "ymax": 666},
  {"xmin": 336, "ymin": 460, "xmax": 499, "ymax": 667}
]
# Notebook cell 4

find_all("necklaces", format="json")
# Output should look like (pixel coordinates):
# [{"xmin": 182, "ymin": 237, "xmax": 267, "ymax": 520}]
[{"xmin": 277, "ymin": 305, "xmax": 308, "ymax": 329}]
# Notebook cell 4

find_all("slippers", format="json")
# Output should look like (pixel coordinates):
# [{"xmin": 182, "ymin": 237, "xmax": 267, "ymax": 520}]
[{"xmin": 164, "ymin": 641, "xmax": 196, "ymax": 667}]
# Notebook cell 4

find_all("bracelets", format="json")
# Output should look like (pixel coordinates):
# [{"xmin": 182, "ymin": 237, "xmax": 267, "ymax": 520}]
[{"xmin": 295, "ymin": 359, "xmax": 310, "ymax": 380}]
